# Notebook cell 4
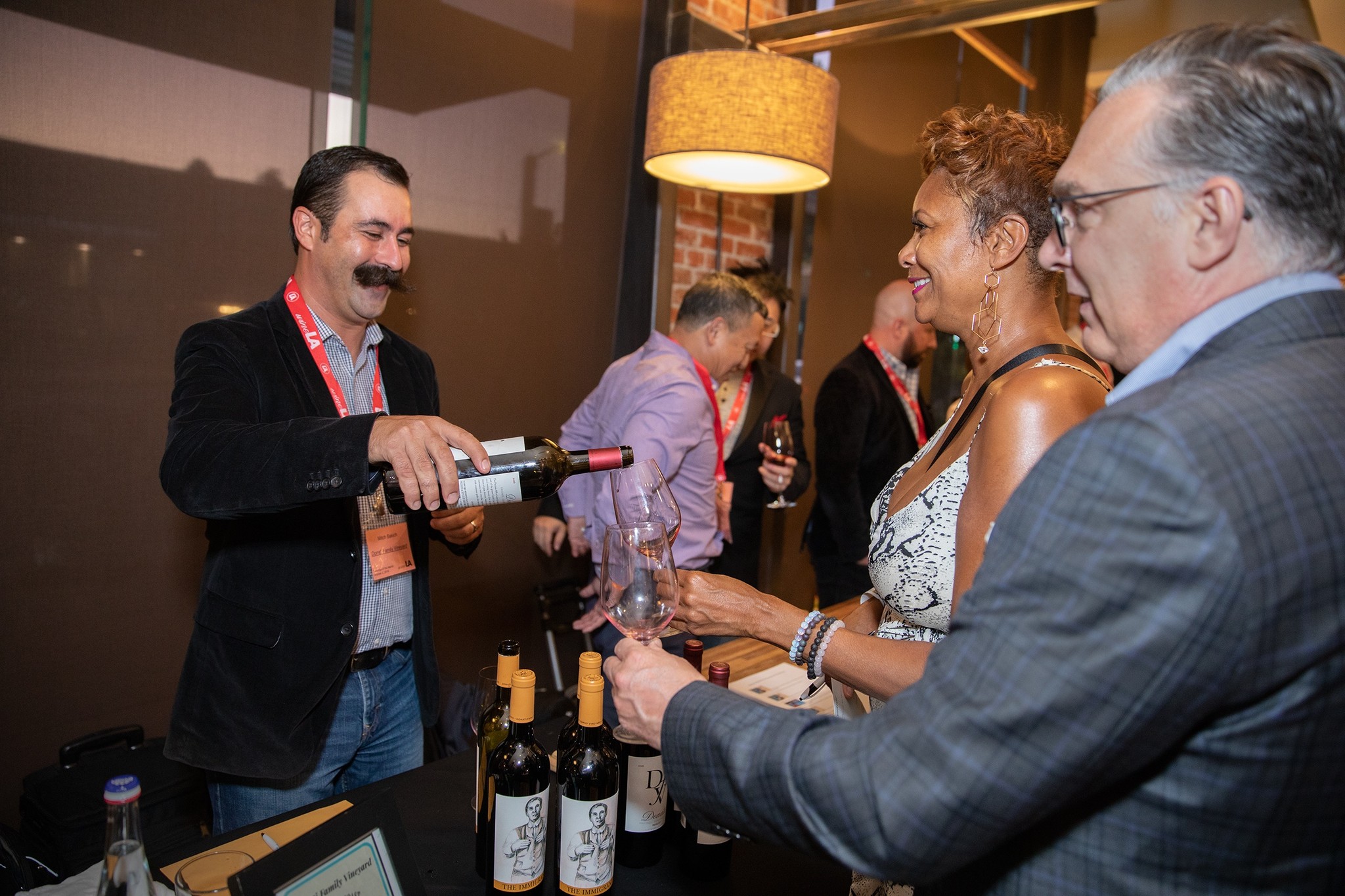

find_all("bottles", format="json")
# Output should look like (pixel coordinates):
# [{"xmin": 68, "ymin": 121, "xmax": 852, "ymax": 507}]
[
  {"xmin": 382, "ymin": 432, "xmax": 635, "ymax": 514},
  {"xmin": 96, "ymin": 775, "xmax": 157, "ymax": 895},
  {"xmin": 472, "ymin": 639, "xmax": 735, "ymax": 895}
]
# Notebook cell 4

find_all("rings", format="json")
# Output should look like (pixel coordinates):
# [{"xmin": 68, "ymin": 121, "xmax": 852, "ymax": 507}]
[{"xmin": 470, "ymin": 520, "xmax": 477, "ymax": 533}]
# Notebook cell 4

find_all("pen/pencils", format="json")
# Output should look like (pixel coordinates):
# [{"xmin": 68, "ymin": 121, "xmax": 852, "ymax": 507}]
[
  {"xmin": 799, "ymin": 629, "xmax": 876, "ymax": 702},
  {"xmin": 261, "ymin": 833, "xmax": 280, "ymax": 852}
]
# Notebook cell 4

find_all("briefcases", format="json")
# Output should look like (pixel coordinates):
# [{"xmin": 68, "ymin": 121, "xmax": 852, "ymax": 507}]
[{"xmin": 21, "ymin": 725, "xmax": 205, "ymax": 889}]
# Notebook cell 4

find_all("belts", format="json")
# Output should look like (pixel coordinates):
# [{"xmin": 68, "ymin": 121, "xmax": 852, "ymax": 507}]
[{"xmin": 345, "ymin": 642, "xmax": 396, "ymax": 673}]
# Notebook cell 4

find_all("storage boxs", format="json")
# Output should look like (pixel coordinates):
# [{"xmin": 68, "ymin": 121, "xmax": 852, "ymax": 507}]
[{"xmin": 18, "ymin": 723, "xmax": 213, "ymax": 888}]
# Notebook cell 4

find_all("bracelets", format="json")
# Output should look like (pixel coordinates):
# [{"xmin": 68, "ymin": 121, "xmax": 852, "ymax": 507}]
[{"xmin": 788, "ymin": 610, "xmax": 846, "ymax": 680}]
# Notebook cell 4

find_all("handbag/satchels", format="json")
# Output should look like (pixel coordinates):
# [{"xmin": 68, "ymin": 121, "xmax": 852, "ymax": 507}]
[{"xmin": 435, "ymin": 679, "xmax": 485, "ymax": 756}]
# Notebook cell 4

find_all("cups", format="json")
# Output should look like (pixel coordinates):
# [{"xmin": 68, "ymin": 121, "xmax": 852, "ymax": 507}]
[{"xmin": 175, "ymin": 851, "xmax": 256, "ymax": 896}]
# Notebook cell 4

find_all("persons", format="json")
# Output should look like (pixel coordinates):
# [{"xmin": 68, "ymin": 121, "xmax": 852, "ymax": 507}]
[
  {"xmin": 158, "ymin": 148, "xmax": 492, "ymax": 844},
  {"xmin": 531, "ymin": 257, "xmax": 813, "ymax": 656},
  {"xmin": 555, "ymin": 272, "xmax": 769, "ymax": 731},
  {"xmin": 648, "ymin": 105, "xmax": 1110, "ymax": 896},
  {"xmin": 603, "ymin": 21, "xmax": 1345, "ymax": 896},
  {"xmin": 804, "ymin": 280, "xmax": 940, "ymax": 613}
]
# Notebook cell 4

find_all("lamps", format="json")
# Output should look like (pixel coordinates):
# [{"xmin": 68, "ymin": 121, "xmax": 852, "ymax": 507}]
[{"xmin": 642, "ymin": 0, "xmax": 840, "ymax": 195}]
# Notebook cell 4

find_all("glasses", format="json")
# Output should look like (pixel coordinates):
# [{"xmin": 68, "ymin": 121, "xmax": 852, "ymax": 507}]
[
  {"xmin": 760, "ymin": 318, "xmax": 781, "ymax": 338},
  {"xmin": 1047, "ymin": 176, "xmax": 1253, "ymax": 249}
]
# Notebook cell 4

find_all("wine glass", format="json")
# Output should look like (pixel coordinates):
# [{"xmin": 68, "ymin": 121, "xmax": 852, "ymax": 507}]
[
  {"xmin": 601, "ymin": 459, "xmax": 681, "ymax": 745},
  {"xmin": 762, "ymin": 421, "xmax": 798, "ymax": 508},
  {"xmin": 470, "ymin": 666, "xmax": 498, "ymax": 810}
]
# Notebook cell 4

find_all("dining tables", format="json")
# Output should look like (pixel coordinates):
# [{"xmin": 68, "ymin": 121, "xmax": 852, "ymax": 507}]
[
  {"xmin": 144, "ymin": 710, "xmax": 734, "ymax": 896},
  {"xmin": 700, "ymin": 594, "xmax": 871, "ymax": 721}
]
